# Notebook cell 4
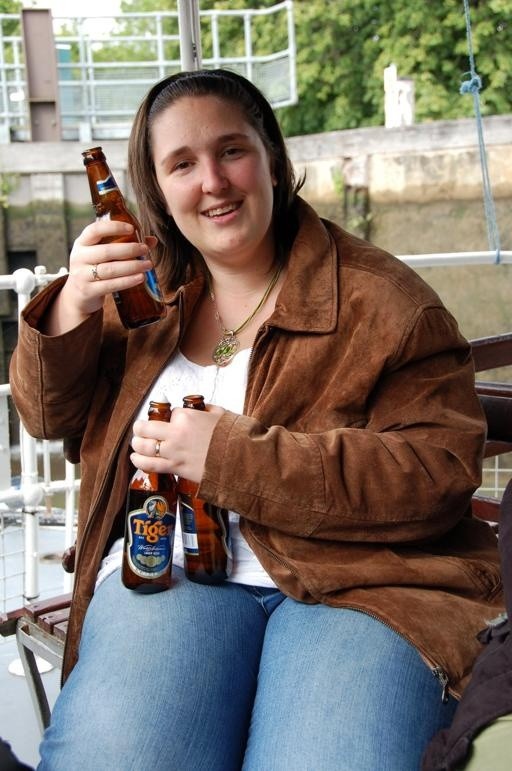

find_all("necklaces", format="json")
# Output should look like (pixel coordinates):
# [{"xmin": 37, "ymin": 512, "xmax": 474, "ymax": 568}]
[{"xmin": 205, "ymin": 231, "xmax": 291, "ymax": 366}]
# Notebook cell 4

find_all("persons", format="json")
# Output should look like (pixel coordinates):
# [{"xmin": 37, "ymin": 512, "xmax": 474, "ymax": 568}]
[{"xmin": 7, "ymin": 68, "xmax": 506, "ymax": 771}]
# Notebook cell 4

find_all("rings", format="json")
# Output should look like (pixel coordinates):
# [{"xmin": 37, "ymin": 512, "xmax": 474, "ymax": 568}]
[
  {"xmin": 90, "ymin": 262, "xmax": 101, "ymax": 282},
  {"xmin": 153, "ymin": 440, "xmax": 162, "ymax": 457}
]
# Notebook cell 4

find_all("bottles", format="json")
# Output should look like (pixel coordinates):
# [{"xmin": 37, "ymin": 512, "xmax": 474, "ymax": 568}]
[
  {"xmin": 81, "ymin": 146, "xmax": 168, "ymax": 331},
  {"xmin": 121, "ymin": 400, "xmax": 179, "ymax": 593},
  {"xmin": 177, "ymin": 393, "xmax": 230, "ymax": 585}
]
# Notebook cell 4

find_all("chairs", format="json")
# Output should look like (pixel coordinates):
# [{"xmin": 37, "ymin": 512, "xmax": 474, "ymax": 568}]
[{"xmin": 0, "ymin": 383, "xmax": 510, "ymax": 737}]
